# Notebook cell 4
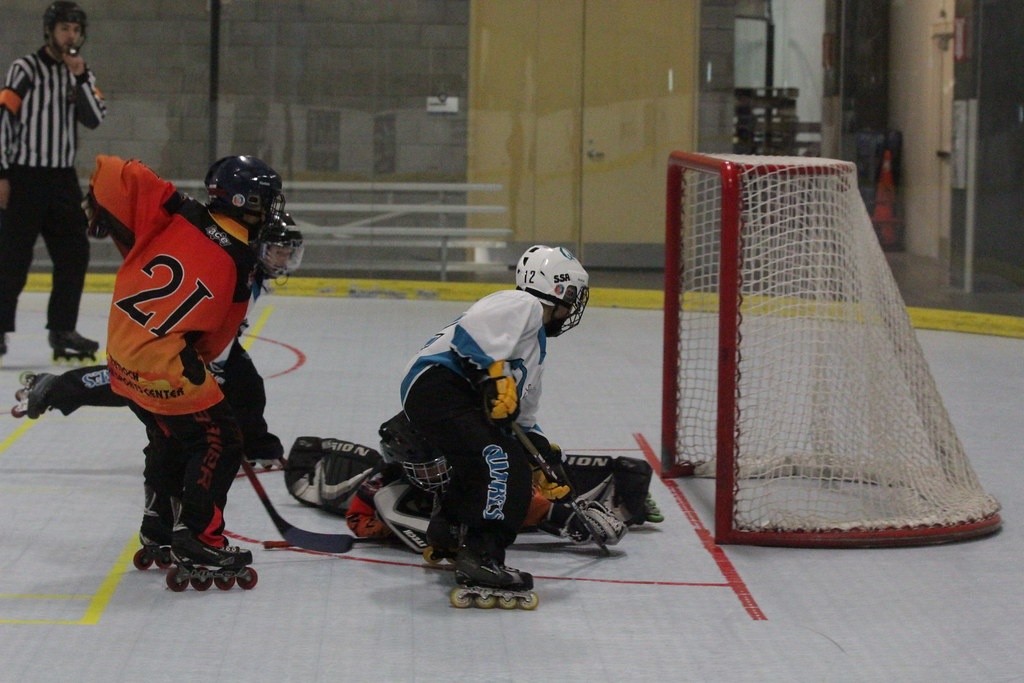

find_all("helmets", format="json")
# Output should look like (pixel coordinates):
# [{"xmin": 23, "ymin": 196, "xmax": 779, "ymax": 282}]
[
  {"xmin": 205, "ymin": 154, "xmax": 281, "ymax": 218},
  {"xmin": 515, "ymin": 244, "xmax": 590, "ymax": 337},
  {"xmin": 252, "ymin": 212, "xmax": 303, "ymax": 275},
  {"xmin": 378, "ymin": 409, "xmax": 453, "ymax": 495},
  {"xmin": 44, "ymin": 1, "xmax": 86, "ymax": 43}
]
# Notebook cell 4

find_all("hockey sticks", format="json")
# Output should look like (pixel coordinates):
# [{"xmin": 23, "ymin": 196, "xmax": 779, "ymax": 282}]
[
  {"xmin": 109, "ymin": 232, "xmax": 356, "ymax": 554},
  {"xmin": 511, "ymin": 419, "xmax": 612, "ymax": 555}
]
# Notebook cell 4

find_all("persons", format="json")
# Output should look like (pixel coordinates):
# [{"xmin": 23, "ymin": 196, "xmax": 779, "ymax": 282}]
[
  {"xmin": 345, "ymin": 412, "xmax": 663, "ymax": 555},
  {"xmin": 398, "ymin": 242, "xmax": 590, "ymax": 610},
  {"xmin": 91, "ymin": 153, "xmax": 285, "ymax": 597},
  {"xmin": 0, "ymin": 0, "xmax": 106, "ymax": 365},
  {"xmin": 11, "ymin": 211, "xmax": 305, "ymax": 471}
]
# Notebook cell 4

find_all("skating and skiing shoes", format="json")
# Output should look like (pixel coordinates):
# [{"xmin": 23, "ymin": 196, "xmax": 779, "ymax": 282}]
[
  {"xmin": 164, "ymin": 480, "xmax": 257, "ymax": 592},
  {"xmin": 446, "ymin": 550, "xmax": 538, "ymax": 609},
  {"xmin": 421, "ymin": 519, "xmax": 463, "ymax": 567},
  {"xmin": 133, "ymin": 483, "xmax": 171, "ymax": 570},
  {"xmin": 47, "ymin": 326, "xmax": 101, "ymax": 363},
  {"xmin": 11, "ymin": 371, "xmax": 61, "ymax": 419}
]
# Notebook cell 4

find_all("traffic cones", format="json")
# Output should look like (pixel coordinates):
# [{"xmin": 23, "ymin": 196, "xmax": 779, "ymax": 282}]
[{"xmin": 871, "ymin": 150, "xmax": 905, "ymax": 251}]
